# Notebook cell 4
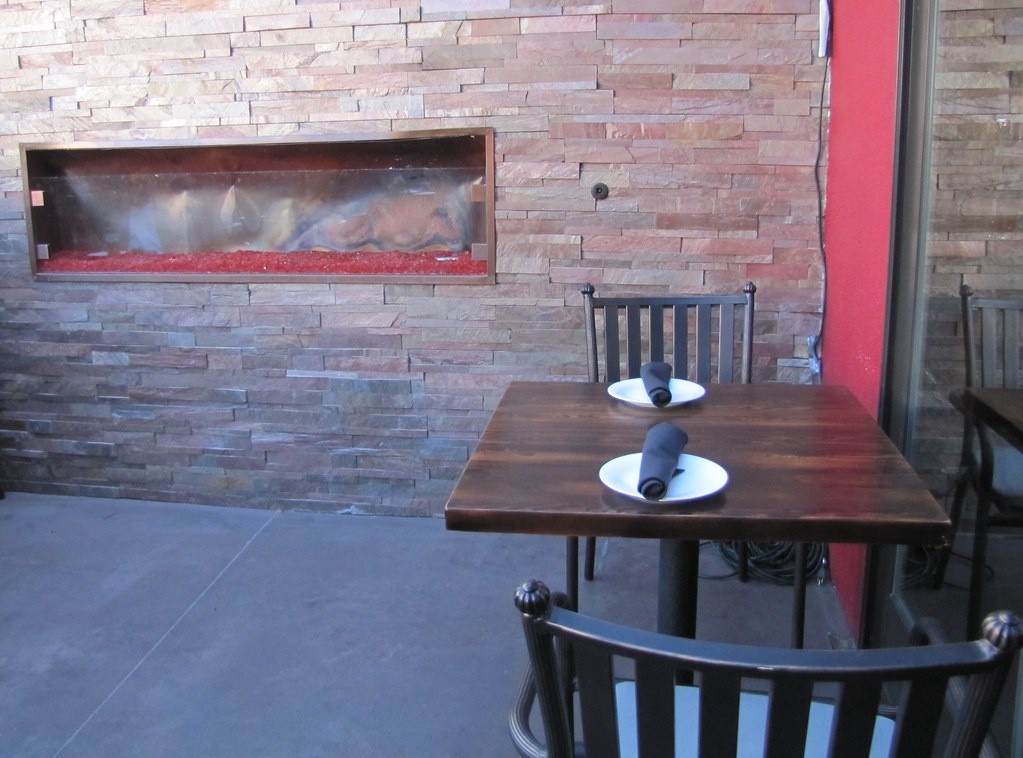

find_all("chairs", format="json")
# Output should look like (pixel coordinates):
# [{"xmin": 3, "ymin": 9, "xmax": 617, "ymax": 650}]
[
  {"xmin": 565, "ymin": 281, "xmax": 756, "ymax": 583},
  {"xmin": 508, "ymin": 579, "xmax": 1023, "ymax": 758},
  {"xmin": 933, "ymin": 285, "xmax": 1023, "ymax": 642}
]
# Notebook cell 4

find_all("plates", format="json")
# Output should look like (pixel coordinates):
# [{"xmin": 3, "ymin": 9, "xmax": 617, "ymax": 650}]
[
  {"xmin": 599, "ymin": 452, "xmax": 730, "ymax": 506},
  {"xmin": 606, "ymin": 377, "xmax": 706, "ymax": 409}
]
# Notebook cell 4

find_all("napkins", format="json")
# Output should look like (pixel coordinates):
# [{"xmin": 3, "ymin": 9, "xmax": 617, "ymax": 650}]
[
  {"xmin": 640, "ymin": 362, "xmax": 672, "ymax": 406},
  {"xmin": 637, "ymin": 422, "xmax": 688, "ymax": 501}
]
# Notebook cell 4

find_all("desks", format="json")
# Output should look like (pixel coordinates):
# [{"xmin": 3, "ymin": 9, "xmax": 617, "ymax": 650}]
[
  {"xmin": 445, "ymin": 381, "xmax": 952, "ymax": 687},
  {"xmin": 962, "ymin": 387, "xmax": 1023, "ymax": 455}
]
